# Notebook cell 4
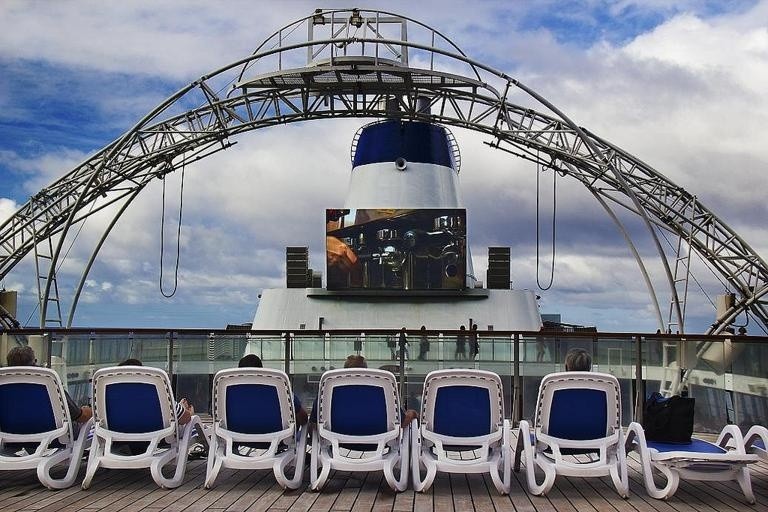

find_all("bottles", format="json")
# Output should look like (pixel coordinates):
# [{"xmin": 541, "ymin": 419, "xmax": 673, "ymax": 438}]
[
  {"xmin": 345, "ymin": 229, "xmax": 354, "ymax": 252},
  {"xmin": 356, "ymin": 226, "xmax": 368, "ymax": 249}
]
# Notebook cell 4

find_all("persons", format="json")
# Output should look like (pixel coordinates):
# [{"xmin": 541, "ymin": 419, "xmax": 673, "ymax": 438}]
[
  {"xmin": 654, "ymin": 329, "xmax": 681, "ymax": 367},
  {"xmin": 468, "ymin": 324, "xmax": 479, "ymax": 361},
  {"xmin": 232, "ymin": 353, "xmax": 308, "ymax": 451},
  {"xmin": 418, "ymin": 325, "xmax": 430, "ymax": 361},
  {"xmin": 455, "ymin": 326, "xmax": 468, "ymax": 362},
  {"xmin": 535, "ymin": 327, "xmax": 547, "ymax": 363},
  {"xmin": 531, "ymin": 347, "xmax": 599, "ymax": 453},
  {"xmin": 4, "ymin": 346, "xmax": 96, "ymax": 452},
  {"xmin": 326, "ymin": 209, "xmax": 443, "ymax": 289},
  {"xmin": 116, "ymin": 358, "xmax": 209, "ymax": 460},
  {"xmin": 308, "ymin": 354, "xmax": 419, "ymax": 458},
  {"xmin": 399, "ymin": 327, "xmax": 411, "ymax": 360}
]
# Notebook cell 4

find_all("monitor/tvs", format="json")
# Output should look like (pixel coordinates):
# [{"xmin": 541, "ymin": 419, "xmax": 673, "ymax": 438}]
[{"xmin": 326, "ymin": 208, "xmax": 467, "ymax": 292}]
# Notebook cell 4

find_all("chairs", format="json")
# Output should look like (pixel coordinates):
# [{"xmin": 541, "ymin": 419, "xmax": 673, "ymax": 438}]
[
  {"xmin": 725, "ymin": 422, "xmax": 767, "ymax": 459},
  {"xmin": 410, "ymin": 367, "xmax": 510, "ymax": 495},
  {"xmin": 0, "ymin": 367, "xmax": 94, "ymax": 493},
  {"xmin": 626, "ymin": 410, "xmax": 759, "ymax": 505},
  {"xmin": 514, "ymin": 372, "xmax": 630, "ymax": 499},
  {"xmin": 309, "ymin": 366, "xmax": 410, "ymax": 492},
  {"xmin": 204, "ymin": 364, "xmax": 308, "ymax": 491},
  {"xmin": 81, "ymin": 366, "xmax": 212, "ymax": 490}
]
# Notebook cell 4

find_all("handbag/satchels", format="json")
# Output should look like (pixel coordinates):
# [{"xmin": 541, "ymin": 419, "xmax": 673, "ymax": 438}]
[{"xmin": 645, "ymin": 392, "xmax": 696, "ymax": 444}]
